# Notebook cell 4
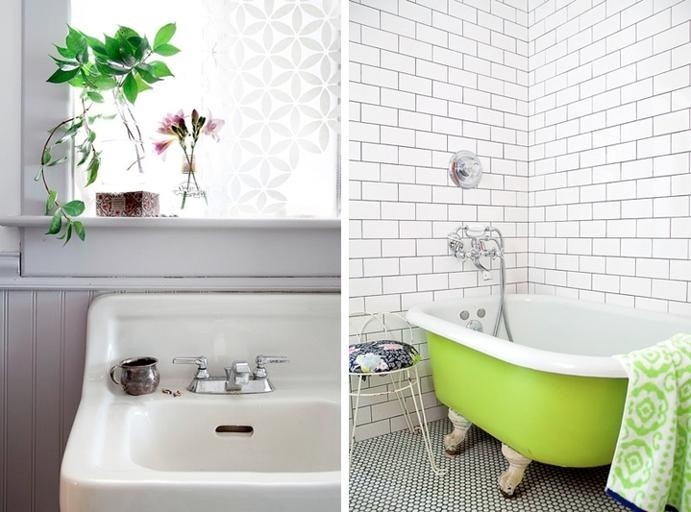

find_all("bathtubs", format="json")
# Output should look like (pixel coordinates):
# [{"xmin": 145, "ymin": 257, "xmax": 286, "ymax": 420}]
[{"xmin": 405, "ymin": 291, "xmax": 691, "ymax": 498}]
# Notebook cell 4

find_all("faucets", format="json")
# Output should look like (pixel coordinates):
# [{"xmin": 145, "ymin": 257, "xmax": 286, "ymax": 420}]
[
  {"xmin": 224, "ymin": 362, "xmax": 254, "ymax": 392},
  {"xmin": 447, "ymin": 222, "xmax": 502, "ymax": 280}
]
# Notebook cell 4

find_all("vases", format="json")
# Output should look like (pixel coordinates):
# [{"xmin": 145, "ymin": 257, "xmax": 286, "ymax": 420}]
[{"xmin": 169, "ymin": 153, "xmax": 212, "ymax": 216}]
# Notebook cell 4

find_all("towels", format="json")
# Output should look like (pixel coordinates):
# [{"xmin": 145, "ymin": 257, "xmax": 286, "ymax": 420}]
[{"xmin": 603, "ymin": 331, "xmax": 691, "ymax": 512}]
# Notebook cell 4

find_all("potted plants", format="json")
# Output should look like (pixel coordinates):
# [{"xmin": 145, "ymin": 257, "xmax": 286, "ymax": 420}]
[{"xmin": 31, "ymin": 12, "xmax": 183, "ymax": 244}]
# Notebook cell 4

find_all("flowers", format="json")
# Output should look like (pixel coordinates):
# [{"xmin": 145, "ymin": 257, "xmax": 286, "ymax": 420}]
[{"xmin": 153, "ymin": 110, "xmax": 227, "ymax": 211}]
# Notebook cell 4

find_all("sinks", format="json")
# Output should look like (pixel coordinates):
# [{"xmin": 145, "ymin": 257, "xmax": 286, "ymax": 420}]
[{"xmin": 60, "ymin": 391, "xmax": 342, "ymax": 512}]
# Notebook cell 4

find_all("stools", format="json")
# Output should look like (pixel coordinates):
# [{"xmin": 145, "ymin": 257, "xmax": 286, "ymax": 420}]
[{"xmin": 348, "ymin": 310, "xmax": 450, "ymax": 479}]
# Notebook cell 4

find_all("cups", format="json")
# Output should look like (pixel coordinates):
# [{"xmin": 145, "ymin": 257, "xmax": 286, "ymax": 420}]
[{"xmin": 110, "ymin": 354, "xmax": 161, "ymax": 398}]
